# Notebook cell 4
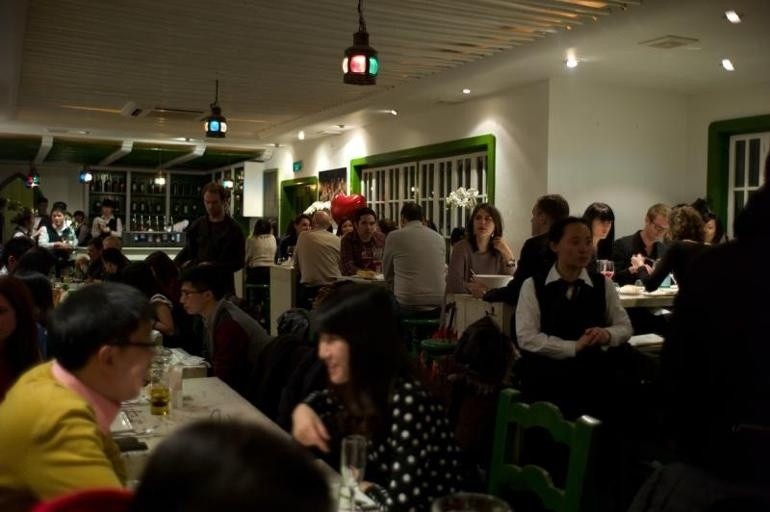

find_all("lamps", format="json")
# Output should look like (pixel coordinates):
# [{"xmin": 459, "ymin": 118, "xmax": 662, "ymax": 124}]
[
  {"xmin": 25, "ymin": 152, "xmax": 40, "ymax": 191},
  {"xmin": 79, "ymin": 163, "xmax": 93, "ymax": 184},
  {"xmin": 154, "ymin": 151, "xmax": 167, "ymax": 188},
  {"xmin": 342, "ymin": 0, "xmax": 379, "ymax": 86}
]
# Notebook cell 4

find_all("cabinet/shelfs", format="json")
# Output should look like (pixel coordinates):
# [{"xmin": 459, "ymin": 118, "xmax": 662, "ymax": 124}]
[{"xmin": 84, "ymin": 161, "xmax": 265, "ymax": 235}]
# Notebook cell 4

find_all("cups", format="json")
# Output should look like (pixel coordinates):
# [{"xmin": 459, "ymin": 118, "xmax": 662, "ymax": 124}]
[
  {"xmin": 167, "ymin": 367, "xmax": 187, "ymax": 409},
  {"xmin": 288, "ymin": 246, "xmax": 295, "ymax": 257},
  {"xmin": 431, "ymin": 490, "xmax": 511, "ymax": 512},
  {"xmin": 599, "ymin": 258, "xmax": 615, "ymax": 278},
  {"xmin": 148, "ymin": 367, "xmax": 172, "ymax": 418},
  {"xmin": 660, "ymin": 277, "xmax": 672, "ymax": 289},
  {"xmin": 636, "ymin": 279, "xmax": 646, "ymax": 293}
]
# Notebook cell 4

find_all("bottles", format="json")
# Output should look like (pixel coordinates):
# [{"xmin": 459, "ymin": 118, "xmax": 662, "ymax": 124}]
[
  {"xmin": 276, "ymin": 256, "xmax": 283, "ymax": 265},
  {"xmin": 68, "ymin": 231, "xmax": 73, "ymax": 246},
  {"xmin": 50, "ymin": 261, "xmax": 100, "ymax": 300},
  {"xmin": 361, "ymin": 256, "xmax": 383, "ymax": 273},
  {"xmin": 132, "ymin": 214, "xmax": 180, "ymax": 243},
  {"xmin": 89, "ymin": 175, "xmax": 197, "ymax": 213}
]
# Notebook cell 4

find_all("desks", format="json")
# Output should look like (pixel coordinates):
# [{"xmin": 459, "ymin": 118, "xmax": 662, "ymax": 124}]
[{"xmin": 52, "ymin": 275, "xmax": 388, "ymax": 511}]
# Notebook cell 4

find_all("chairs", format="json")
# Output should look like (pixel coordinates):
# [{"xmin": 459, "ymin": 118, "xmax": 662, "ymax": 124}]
[
  {"xmin": 488, "ymin": 388, "xmax": 603, "ymax": 510},
  {"xmin": 244, "ymin": 265, "xmax": 270, "ymax": 310}
]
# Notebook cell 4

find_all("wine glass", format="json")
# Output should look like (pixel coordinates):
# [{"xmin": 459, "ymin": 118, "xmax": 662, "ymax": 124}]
[{"xmin": 340, "ymin": 435, "xmax": 368, "ymax": 512}]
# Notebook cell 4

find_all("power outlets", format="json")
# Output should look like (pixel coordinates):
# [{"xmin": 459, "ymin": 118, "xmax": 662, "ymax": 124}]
[{"xmin": 200, "ymin": 79, "xmax": 228, "ymax": 138}]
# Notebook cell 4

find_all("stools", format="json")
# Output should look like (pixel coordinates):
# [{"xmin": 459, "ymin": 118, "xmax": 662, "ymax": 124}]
[
  {"xmin": 397, "ymin": 318, "xmax": 441, "ymax": 365},
  {"xmin": 419, "ymin": 335, "xmax": 458, "ymax": 367}
]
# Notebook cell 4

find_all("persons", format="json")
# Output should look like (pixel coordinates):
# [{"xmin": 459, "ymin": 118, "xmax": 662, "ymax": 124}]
[{"xmin": 1, "ymin": 182, "xmax": 770, "ymax": 511}]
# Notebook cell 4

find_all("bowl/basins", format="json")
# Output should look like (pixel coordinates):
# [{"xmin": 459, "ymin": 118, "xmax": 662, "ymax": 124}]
[{"xmin": 473, "ymin": 273, "xmax": 514, "ymax": 289}]
